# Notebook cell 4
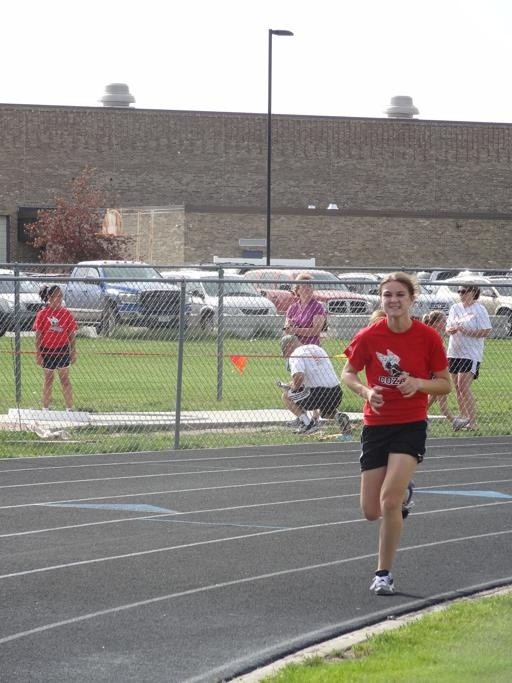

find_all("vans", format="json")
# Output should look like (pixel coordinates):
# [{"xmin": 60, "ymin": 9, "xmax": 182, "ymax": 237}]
[{"xmin": 152, "ymin": 270, "xmax": 278, "ymax": 316}]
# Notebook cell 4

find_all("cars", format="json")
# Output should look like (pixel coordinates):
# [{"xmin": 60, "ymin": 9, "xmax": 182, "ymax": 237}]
[{"xmin": 0, "ymin": 266, "xmax": 512, "ymax": 339}]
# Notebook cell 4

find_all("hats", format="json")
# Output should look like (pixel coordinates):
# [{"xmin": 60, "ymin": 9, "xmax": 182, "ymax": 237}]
[{"xmin": 278, "ymin": 334, "xmax": 297, "ymax": 357}]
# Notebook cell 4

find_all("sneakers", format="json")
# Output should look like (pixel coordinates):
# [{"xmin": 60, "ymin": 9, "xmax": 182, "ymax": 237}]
[
  {"xmin": 402, "ymin": 480, "xmax": 416, "ymax": 520},
  {"xmin": 452, "ymin": 417, "xmax": 480, "ymax": 432},
  {"xmin": 335, "ymin": 411, "xmax": 352, "ymax": 436},
  {"xmin": 369, "ymin": 569, "xmax": 397, "ymax": 598},
  {"xmin": 291, "ymin": 418, "xmax": 321, "ymax": 437}
]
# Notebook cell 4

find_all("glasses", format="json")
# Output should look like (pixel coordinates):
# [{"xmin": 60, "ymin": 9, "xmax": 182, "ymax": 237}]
[{"xmin": 457, "ymin": 288, "xmax": 473, "ymax": 295}]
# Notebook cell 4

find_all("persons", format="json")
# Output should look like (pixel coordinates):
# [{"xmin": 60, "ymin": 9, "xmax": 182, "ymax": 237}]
[
  {"xmin": 341, "ymin": 273, "xmax": 452, "ymax": 594},
  {"xmin": 280, "ymin": 273, "xmax": 352, "ymax": 436},
  {"xmin": 422, "ymin": 281, "xmax": 492, "ymax": 432},
  {"xmin": 33, "ymin": 285, "xmax": 79, "ymax": 412}
]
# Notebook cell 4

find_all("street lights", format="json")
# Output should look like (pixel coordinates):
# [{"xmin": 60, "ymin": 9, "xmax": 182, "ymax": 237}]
[{"xmin": 266, "ymin": 28, "xmax": 294, "ymax": 266}]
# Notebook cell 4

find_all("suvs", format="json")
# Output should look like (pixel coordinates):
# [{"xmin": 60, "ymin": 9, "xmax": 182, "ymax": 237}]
[
  {"xmin": 64, "ymin": 260, "xmax": 192, "ymax": 329},
  {"xmin": 242, "ymin": 269, "xmax": 375, "ymax": 316}
]
